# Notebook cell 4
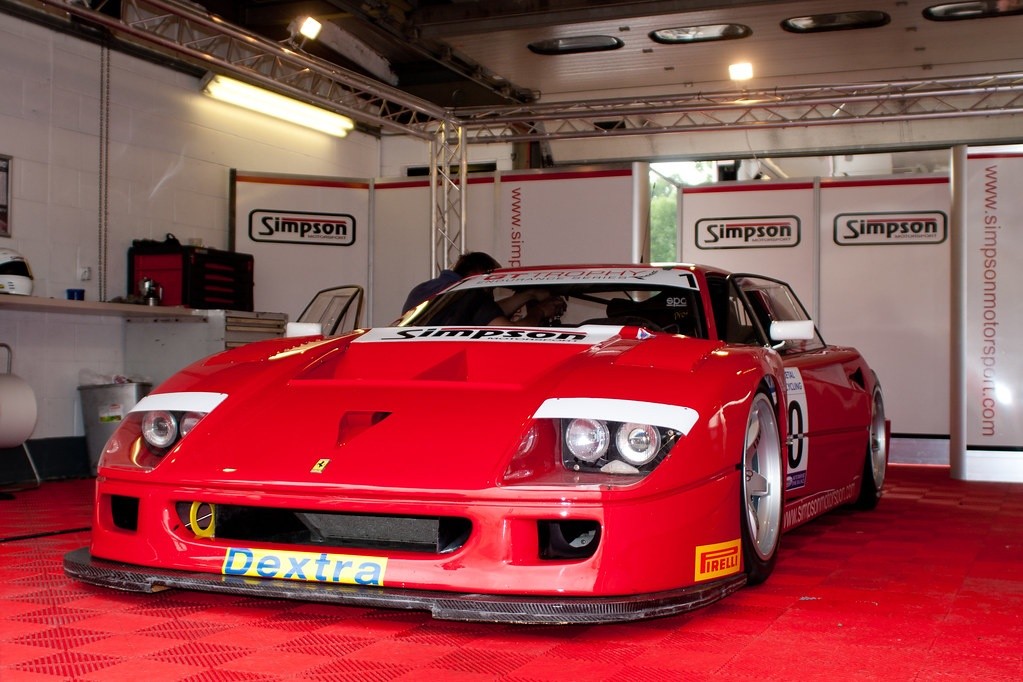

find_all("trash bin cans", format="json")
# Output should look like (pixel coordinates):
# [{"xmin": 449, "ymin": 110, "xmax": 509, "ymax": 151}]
[{"xmin": 76, "ymin": 382, "xmax": 152, "ymax": 477}]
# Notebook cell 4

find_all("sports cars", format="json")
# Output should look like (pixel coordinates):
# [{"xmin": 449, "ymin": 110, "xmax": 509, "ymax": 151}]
[{"xmin": 64, "ymin": 260, "xmax": 890, "ymax": 627}]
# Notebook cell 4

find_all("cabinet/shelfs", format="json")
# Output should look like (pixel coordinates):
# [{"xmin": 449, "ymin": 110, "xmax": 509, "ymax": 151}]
[{"xmin": 0, "ymin": 292, "xmax": 289, "ymax": 389}]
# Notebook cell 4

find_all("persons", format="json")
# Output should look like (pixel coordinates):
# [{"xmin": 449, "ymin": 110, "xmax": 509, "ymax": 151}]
[
  {"xmin": 604, "ymin": 274, "xmax": 809, "ymax": 356},
  {"xmin": 399, "ymin": 246, "xmax": 555, "ymax": 327}
]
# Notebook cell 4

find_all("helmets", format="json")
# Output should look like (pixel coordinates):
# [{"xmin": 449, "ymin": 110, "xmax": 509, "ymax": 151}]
[{"xmin": 0, "ymin": 247, "xmax": 34, "ymax": 296}]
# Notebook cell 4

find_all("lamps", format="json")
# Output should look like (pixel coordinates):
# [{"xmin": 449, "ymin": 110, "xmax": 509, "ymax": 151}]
[
  {"xmin": 287, "ymin": 17, "xmax": 323, "ymax": 41},
  {"xmin": 197, "ymin": 69, "xmax": 356, "ymax": 139}
]
